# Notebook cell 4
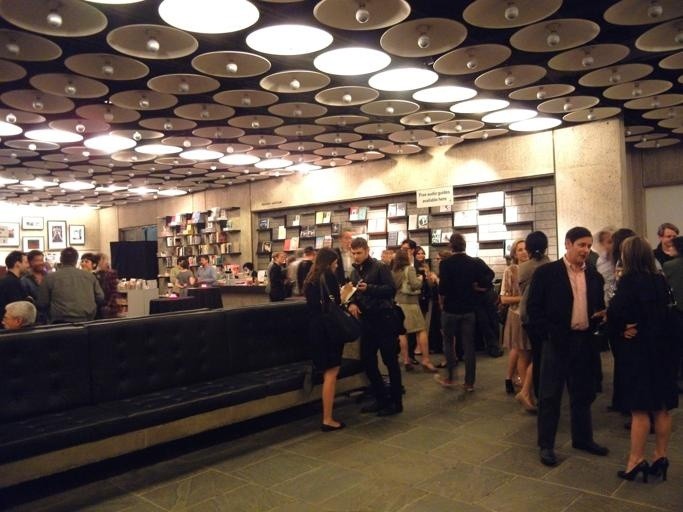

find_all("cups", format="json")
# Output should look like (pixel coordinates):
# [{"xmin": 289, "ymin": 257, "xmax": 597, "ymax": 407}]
[
  {"xmin": 225, "ymin": 274, "xmax": 230, "ymax": 284},
  {"xmin": 263, "ymin": 275, "xmax": 267, "ymax": 285}
]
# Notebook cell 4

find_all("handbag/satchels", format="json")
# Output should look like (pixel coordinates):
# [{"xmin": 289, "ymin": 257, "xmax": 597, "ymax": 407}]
[
  {"xmin": 372, "ymin": 307, "xmax": 405, "ymax": 335},
  {"xmin": 496, "ymin": 299, "xmax": 509, "ymax": 325},
  {"xmin": 401, "ymin": 280, "xmax": 421, "ymax": 296},
  {"xmin": 320, "ymin": 295, "xmax": 359, "ymax": 343}
]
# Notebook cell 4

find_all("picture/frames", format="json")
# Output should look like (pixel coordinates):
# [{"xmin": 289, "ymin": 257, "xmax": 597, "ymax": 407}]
[
  {"xmin": 0, "ymin": 222, "xmax": 19, "ymax": 247},
  {"xmin": 69, "ymin": 224, "xmax": 85, "ymax": 245},
  {"xmin": 47, "ymin": 220, "xmax": 67, "ymax": 251},
  {"xmin": 22, "ymin": 215, "xmax": 44, "ymax": 230},
  {"xmin": 21, "ymin": 236, "xmax": 44, "ymax": 253}
]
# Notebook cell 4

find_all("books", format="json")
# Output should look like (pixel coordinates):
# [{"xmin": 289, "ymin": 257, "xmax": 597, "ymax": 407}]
[
  {"xmin": 258, "ymin": 192, "xmax": 537, "ymax": 281},
  {"xmin": 167, "ymin": 208, "xmax": 232, "ymax": 275}
]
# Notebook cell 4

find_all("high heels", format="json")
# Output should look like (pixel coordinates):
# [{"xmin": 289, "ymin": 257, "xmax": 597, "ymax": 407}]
[
  {"xmin": 649, "ymin": 456, "xmax": 668, "ymax": 481},
  {"xmin": 618, "ymin": 459, "xmax": 649, "ymax": 483}
]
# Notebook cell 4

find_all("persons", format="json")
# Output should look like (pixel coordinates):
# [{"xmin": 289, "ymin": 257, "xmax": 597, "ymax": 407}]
[
  {"xmin": 266, "ymin": 246, "xmax": 319, "ymax": 300},
  {"xmin": 170, "ymin": 254, "xmax": 217, "ymax": 294},
  {"xmin": 304, "ymin": 247, "xmax": 353, "ymax": 431},
  {"xmin": 603, "ymin": 235, "xmax": 682, "ymax": 482},
  {"xmin": 52, "ymin": 227, "xmax": 62, "ymax": 242},
  {"xmin": 381, "ymin": 224, "xmax": 682, "ymax": 436},
  {"xmin": 347, "ymin": 237, "xmax": 406, "ymax": 417},
  {"xmin": 523, "ymin": 225, "xmax": 608, "ymax": 467},
  {"xmin": 0, "ymin": 246, "xmax": 120, "ymax": 334}
]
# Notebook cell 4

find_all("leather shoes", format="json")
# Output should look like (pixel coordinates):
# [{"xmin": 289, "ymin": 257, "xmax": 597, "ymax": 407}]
[
  {"xmin": 362, "ymin": 351, "xmax": 537, "ymax": 416},
  {"xmin": 572, "ymin": 440, "xmax": 608, "ymax": 456},
  {"xmin": 303, "ymin": 364, "xmax": 312, "ymax": 393},
  {"xmin": 322, "ymin": 421, "xmax": 346, "ymax": 432},
  {"xmin": 541, "ymin": 448, "xmax": 558, "ymax": 466}
]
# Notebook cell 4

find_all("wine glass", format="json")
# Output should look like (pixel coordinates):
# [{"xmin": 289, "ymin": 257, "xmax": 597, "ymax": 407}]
[{"xmin": 167, "ymin": 283, "xmax": 172, "ymax": 297}]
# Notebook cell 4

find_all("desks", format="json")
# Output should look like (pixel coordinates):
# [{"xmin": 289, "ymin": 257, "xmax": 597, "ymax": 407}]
[
  {"xmin": 147, "ymin": 292, "xmax": 205, "ymax": 318},
  {"xmin": 186, "ymin": 284, "xmax": 224, "ymax": 310}
]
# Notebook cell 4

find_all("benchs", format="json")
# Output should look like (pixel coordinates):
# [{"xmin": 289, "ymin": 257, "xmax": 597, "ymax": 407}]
[{"xmin": 0, "ymin": 296, "xmax": 385, "ymax": 486}]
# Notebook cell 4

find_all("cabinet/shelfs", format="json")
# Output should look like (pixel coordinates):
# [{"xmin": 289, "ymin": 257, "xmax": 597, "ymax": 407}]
[
  {"xmin": 115, "ymin": 286, "xmax": 158, "ymax": 319},
  {"xmin": 155, "ymin": 207, "xmax": 240, "ymax": 286},
  {"xmin": 253, "ymin": 186, "xmax": 535, "ymax": 270}
]
